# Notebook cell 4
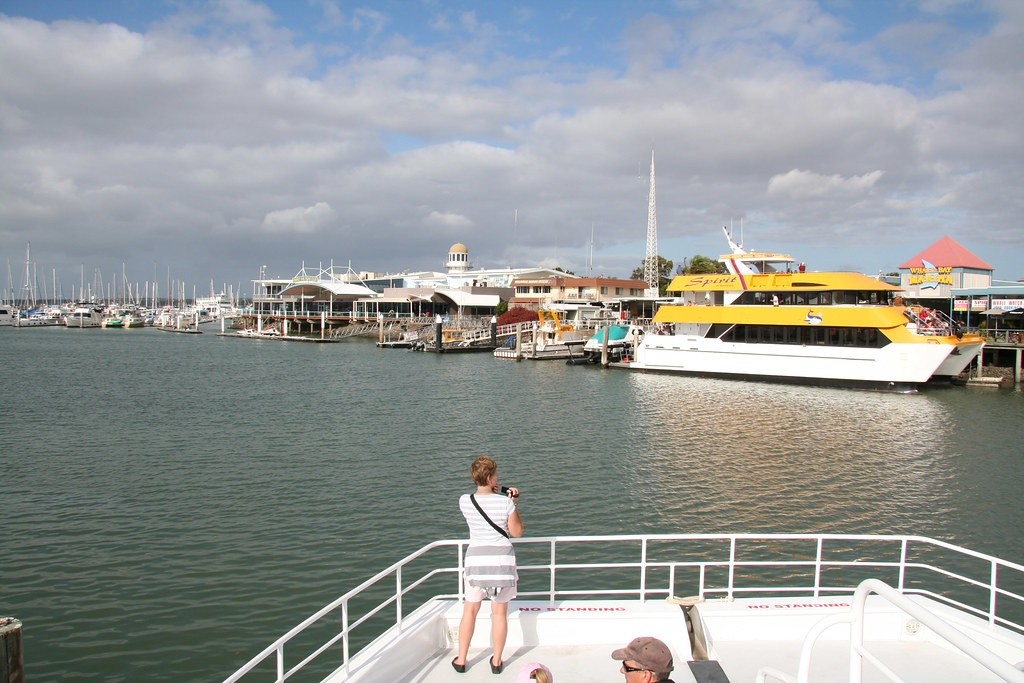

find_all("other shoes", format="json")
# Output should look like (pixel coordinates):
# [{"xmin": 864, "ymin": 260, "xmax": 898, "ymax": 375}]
[
  {"xmin": 490, "ymin": 655, "xmax": 502, "ymax": 674},
  {"xmin": 452, "ymin": 656, "xmax": 466, "ymax": 673}
]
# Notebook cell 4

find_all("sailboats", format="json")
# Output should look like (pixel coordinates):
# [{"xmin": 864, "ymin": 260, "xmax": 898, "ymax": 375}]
[{"xmin": 0, "ymin": 238, "xmax": 243, "ymax": 324}]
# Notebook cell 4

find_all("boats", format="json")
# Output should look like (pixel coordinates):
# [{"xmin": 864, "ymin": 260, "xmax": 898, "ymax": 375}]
[
  {"xmin": 39, "ymin": 309, "xmax": 74, "ymax": 325},
  {"xmin": 237, "ymin": 328, "xmax": 258, "ymax": 334},
  {"xmin": 123, "ymin": 305, "xmax": 146, "ymax": 327},
  {"xmin": 20, "ymin": 314, "xmax": 46, "ymax": 326},
  {"xmin": 610, "ymin": 214, "xmax": 987, "ymax": 391},
  {"xmin": 103, "ymin": 309, "xmax": 125, "ymax": 328},
  {"xmin": 493, "ymin": 307, "xmax": 589, "ymax": 361},
  {"xmin": 64, "ymin": 301, "xmax": 102, "ymax": 327},
  {"xmin": 261, "ymin": 327, "xmax": 280, "ymax": 336},
  {"xmin": 583, "ymin": 310, "xmax": 645, "ymax": 365}
]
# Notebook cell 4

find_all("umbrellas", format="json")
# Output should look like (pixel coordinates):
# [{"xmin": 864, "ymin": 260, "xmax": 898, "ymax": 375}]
[{"xmin": 979, "ymin": 306, "xmax": 1024, "ymax": 336}]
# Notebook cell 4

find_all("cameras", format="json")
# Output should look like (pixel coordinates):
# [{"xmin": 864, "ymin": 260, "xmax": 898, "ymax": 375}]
[{"xmin": 501, "ymin": 487, "xmax": 513, "ymax": 498}]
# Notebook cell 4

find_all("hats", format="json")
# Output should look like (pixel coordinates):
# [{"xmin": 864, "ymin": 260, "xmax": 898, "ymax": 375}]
[
  {"xmin": 611, "ymin": 638, "xmax": 675, "ymax": 672},
  {"xmin": 515, "ymin": 662, "xmax": 552, "ymax": 683}
]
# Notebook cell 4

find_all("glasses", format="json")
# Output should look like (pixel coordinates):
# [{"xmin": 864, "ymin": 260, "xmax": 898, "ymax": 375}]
[{"xmin": 623, "ymin": 660, "xmax": 655, "ymax": 674}]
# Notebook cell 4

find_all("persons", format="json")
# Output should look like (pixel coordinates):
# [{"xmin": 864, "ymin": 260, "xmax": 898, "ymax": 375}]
[
  {"xmin": 893, "ymin": 294, "xmax": 902, "ymax": 305},
  {"xmin": 919, "ymin": 308, "xmax": 951, "ymax": 336},
  {"xmin": 611, "ymin": 636, "xmax": 676, "ymax": 683},
  {"xmin": 770, "ymin": 293, "xmax": 779, "ymax": 307},
  {"xmin": 451, "ymin": 457, "xmax": 525, "ymax": 674},
  {"xmin": 704, "ymin": 298, "xmax": 713, "ymax": 306},
  {"xmin": 687, "ymin": 299, "xmax": 697, "ymax": 306},
  {"xmin": 799, "ymin": 262, "xmax": 805, "ymax": 273},
  {"xmin": 652, "ymin": 326, "xmax": 672, "ymax": 336}
]
